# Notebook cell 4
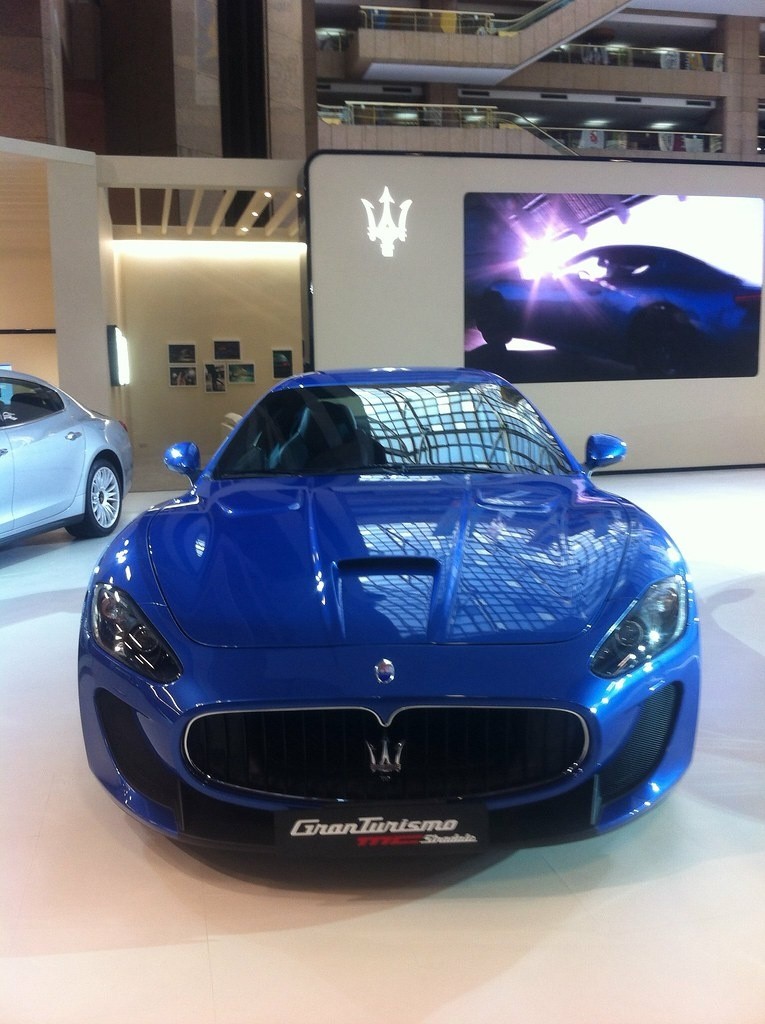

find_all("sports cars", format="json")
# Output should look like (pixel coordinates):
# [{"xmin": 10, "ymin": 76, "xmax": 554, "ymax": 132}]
[{"xmin": 75, "ymin": 364, "xmax": 707, "ymax": 854}]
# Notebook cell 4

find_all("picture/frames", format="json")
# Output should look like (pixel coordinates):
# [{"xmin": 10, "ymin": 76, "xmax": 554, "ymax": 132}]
[
  {"xmin": 167, "ymin": 343, "xmax": 197, "ymax": 365},
  {"xmin": 213, "ymin": 340, "xmax": 241, "ymax": 361},
  {"xmin": 168, "ymin": 366, "xmax": 198, "ymax": 387},
  {"xmin": 204, "ymin": 363, "xmax": 227, "ymax": 393},
  {"xmin": 272, "ymin": 349, "xmax": 293, "ymax": 380},
  {"xmin": 228, "ymin": 363, "xmax": 256, "ymax": 384}
]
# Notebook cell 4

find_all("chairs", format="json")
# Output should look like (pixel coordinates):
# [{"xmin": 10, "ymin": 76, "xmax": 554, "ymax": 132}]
[
  {"xmin": 274, "ymin": 403, "xmax": 374, "ymax": 478},
  {"xmin": 11, "ymin": 392, "xmax": 43, "ymax": 420}
]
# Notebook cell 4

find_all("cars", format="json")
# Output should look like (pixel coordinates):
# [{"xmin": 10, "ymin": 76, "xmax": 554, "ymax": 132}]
[
  {"xmin": 476, "ymin": 245, "xmax": 762, "ymax": 379},
  {"xmin": 1, "ymin": 367, "xmax": 135, "ymax": 547}
]
